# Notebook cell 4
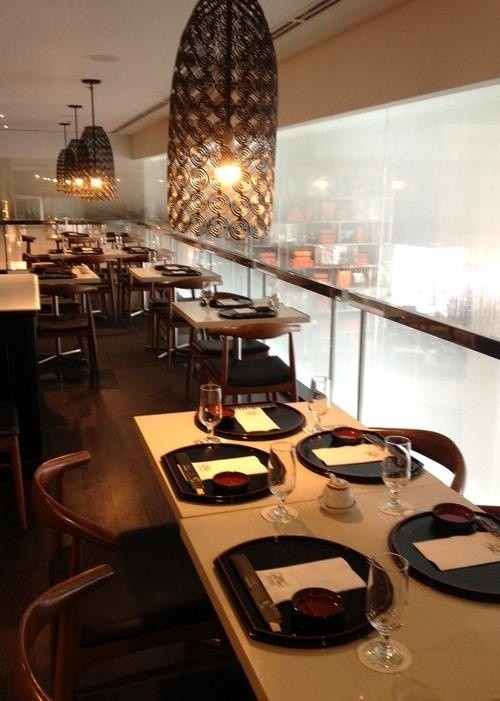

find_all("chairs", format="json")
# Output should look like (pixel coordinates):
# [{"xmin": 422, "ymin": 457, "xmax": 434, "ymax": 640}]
[
  {"xmin": 476, "ymin": 505, "xmax": 500, "ymax": 517},
  {"xmin": 368, "ymin": 427, "xmax": 466, "ymax": 493},
  {"xmin": 31, "ymin": 449, "xmax": 214, "ymax": 680},
  {"xmin": 15, "ymin": 563, "xmax": 255, "ymax": 701}
]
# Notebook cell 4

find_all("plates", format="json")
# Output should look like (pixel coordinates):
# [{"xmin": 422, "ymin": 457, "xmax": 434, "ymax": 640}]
[
  {"xmin": 330, "ymin": 425, "xmax": 365, "ymax": 444},
  {"xmin": 290, "ymin": 588, "xmax": 345, "ymax": 630},
  {"xmin": 212, "ymin": 468, "xmax": 248, "ymax": 493},
  {"xmin": 203, "ymin": 402, "xmax": 234, "ymax": 425},
  {"xmin": 214, "ymin": 531, "xmax": 394, "ymax": 650},
  {"xmin": 315, "ymin": 493, "xmax": 360, "ymax": 516},
  {"xmin": 30, "ymin": 231, "xmax": 276, "ymax": 320},
  {"xmin": 197, "ymin": 400, "xmax": 306, "ymax": 444},
  {"xmin": 165, "ymin": 442, "xmax": 285, "ymax": 507},
  {"xmin": 297, "ymin": 430, "xmax": 423, "ymax": 483},
  {"xmin": 431, "ymin": 500, "xmax": 477, "ymax": 531},
  {"xmin": 392, "ymin": 503, "xmax": 500, "ymax": 605}
]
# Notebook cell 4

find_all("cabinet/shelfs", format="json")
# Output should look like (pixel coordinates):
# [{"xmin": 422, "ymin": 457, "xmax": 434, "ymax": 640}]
[{"xmin": 236, "ymin": 168, "xmax": 388, "ymax": 298}]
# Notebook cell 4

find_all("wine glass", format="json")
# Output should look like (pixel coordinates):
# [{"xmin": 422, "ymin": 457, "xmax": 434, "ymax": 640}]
[
  {"xmin": 198, "ymin": 383, "xmax": 225, "ymax": 447},
  {"xmin": 260, "ymin": 440, "xmax": 299, "ymax": 526},
  {"xmin": 358, "ymin": 551, "xmax": 415, "ymax": 672},
  {"xmin": 378, "ymin": 434, "xmax": 416, "ymax": 517},
  {"xmin": 300, "ymin": 377, "xmax": 332, "ymax": 435}
]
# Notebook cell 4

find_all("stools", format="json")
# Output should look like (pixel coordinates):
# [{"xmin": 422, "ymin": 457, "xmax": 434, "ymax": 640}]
[{"xmin": 0, "ymin": 402, "xmax": 27, "ymax": 530}]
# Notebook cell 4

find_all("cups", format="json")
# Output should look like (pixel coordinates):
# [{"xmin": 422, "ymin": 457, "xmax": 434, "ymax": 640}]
[{"xmin": 324, "ymin": 476, "xmax": 355, "ymax": 508}]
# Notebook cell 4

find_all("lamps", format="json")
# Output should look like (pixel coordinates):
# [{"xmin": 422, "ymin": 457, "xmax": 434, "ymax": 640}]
[
  {"xmin": 165, "ymin": 0, "xmax": 278, "ymax": 241},
  {"xmin": 79, "ymin": 78, "xmax": 119, "ymax": 203},
  {"xmin": 56, "ymin": 123, "xmax": 75, "ymax": 193},
  {"xmin": 64, "ymin": 104, "xmax": 90, "ymax": 199}
]
